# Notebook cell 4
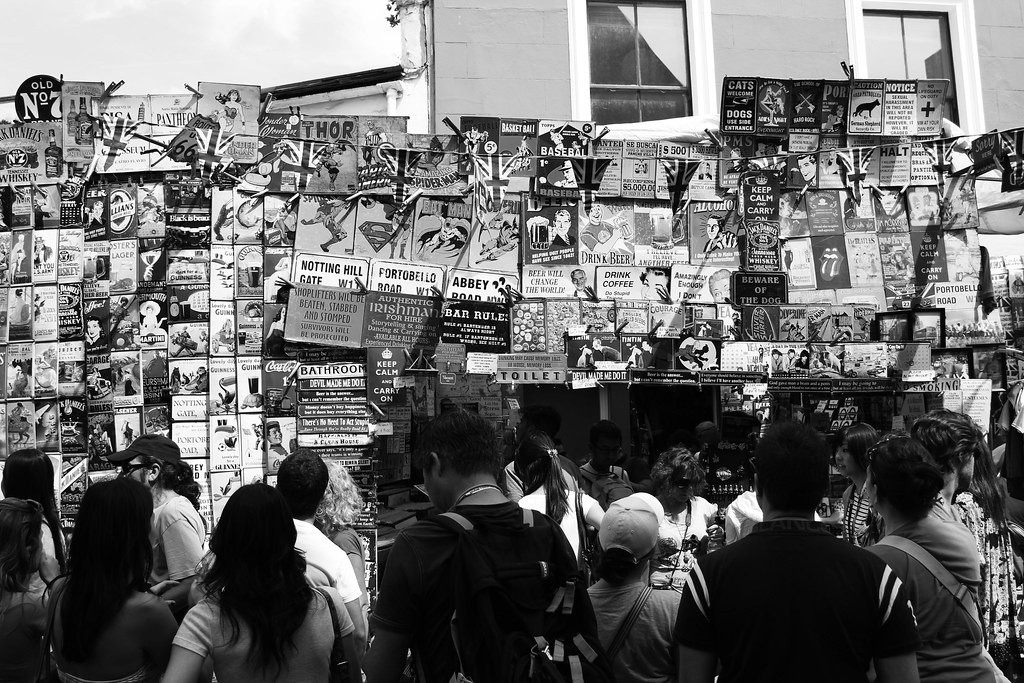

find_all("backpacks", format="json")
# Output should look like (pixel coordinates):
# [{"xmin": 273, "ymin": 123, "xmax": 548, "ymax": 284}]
[{"xmin": 420, "ymin": 508, "xmax": 614, "ymax": 683}]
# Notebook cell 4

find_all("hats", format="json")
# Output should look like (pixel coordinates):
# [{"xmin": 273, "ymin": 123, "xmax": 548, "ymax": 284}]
[
  {"xmin": 106, "ymin": 434, "xmax": 183, "ymax": 464},
  {"xmin": 599, "ymin": 491, "xmax": 664, "ymax": 560}
]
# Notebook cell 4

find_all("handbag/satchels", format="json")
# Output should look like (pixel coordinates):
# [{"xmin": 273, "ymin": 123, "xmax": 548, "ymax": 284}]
[{"xmin": 575, "ymin": 492, "xmax": 600, "ymax": 588}]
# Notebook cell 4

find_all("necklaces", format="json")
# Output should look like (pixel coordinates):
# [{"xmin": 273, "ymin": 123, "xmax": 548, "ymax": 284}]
[{"xmin": 451, "ymin": 483, "xmax": 504, "ymax": 509}]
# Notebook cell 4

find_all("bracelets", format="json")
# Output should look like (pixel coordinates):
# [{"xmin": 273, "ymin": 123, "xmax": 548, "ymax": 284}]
[{"xmin": 46, "ymin": 573, "xmax": 65, "ymax": 588}]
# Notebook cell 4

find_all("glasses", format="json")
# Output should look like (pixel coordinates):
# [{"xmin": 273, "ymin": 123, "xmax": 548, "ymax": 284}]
[
  {"xmin": 515, "ymin": 422, "xmax": 532, "ymax": 428},
  {"xmin": 669, "ymin": 477, "xmax": 700, "ymax": 489},
  {"xmin": 119, "ymin": 462, "xmax": 150, "ymax": 473},
  {"xmin": 592, "ymin": 446, "xmax": 623, "ymax": 454},
  {"xmin": 17, "ymin": 498, "xmax": 44, "ymax": 512}
]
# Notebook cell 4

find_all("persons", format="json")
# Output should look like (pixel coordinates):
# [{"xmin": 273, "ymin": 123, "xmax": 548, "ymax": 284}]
[
  {"xmin": 1, "ymin": 448, "xmax": 69, "ymax": 585},
  {"xmin": 0, "ymin": 496, "xmax": 69, "ymax": 683},
  {"xmin": 587, "ymin": 491, "xmax": 684, "ymax": 683},
  {"xmin": 359, "ymin": 406, "xmax": 599, "ymax": 683},
  {"xmin": 671, "ymin": 420, "xmax": 924, "ymax": 683},
  {"xmin": 274, "ymin": 446, "xmax": 369, "ymax": 674},
  {"xmin": 911, "ymin": 407, "xmax": 985, "ymax": 579},
  {"xmin": 106, "ymin": 435, "xmax": 215, "ymax": 683},
  {"xmin": 43, "ymin": 478, "xmax": 180, "ymax": 683},
  {"xmin": 831, "ymin": 423, "xmax": 887, "ymax": 547},
  {"xmin": 503, "ymin": 400, "xmax": 765, "ymax": 592},
  {"xmin": 157, "ymin": 484, "xmax": 364, "ymax": 683},
  {"xmin": 312, "ymin": 455, "xmax": 365, "ymax": 578},
  {"xmin": 858, "ymin": 436, "xmax": 1010, "ymax": 683},
  {"xmin": 953, "ymin": 431, "xmax": 1024, "ymax": 683}
]
